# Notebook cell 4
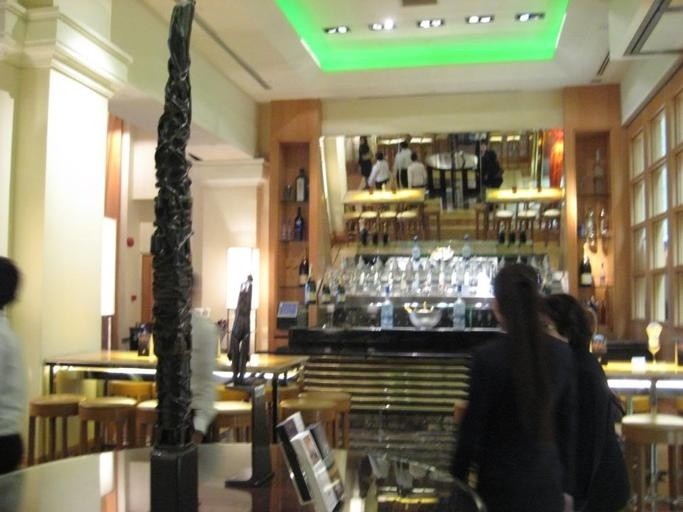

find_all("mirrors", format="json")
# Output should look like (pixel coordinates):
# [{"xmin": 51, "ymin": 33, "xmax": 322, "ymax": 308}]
[{"xmin": 318, "ymin": 132, "xmax": 566, "ymax": 322}]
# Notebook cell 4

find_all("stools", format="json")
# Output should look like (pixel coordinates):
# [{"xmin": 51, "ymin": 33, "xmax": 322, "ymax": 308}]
[{"xmin": 334, "ymin": 132, "xmax": 561, "ymax": 248}]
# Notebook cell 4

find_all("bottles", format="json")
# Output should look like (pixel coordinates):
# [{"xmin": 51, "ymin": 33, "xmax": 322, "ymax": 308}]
[
  {"xmin": 580, "ymin": 147, "xmax": 608, "ymax": 286},
  {"xmin": 453, "ymin": 284, "xmax": 468, "ymax": 330},
  {"xmin": 305, "ymin": 280, "xmax": 344, "ymax": 326},
  {"xmin": 463, "ymin": 237, "xmax": 472, "ymax": 261},
  {"xmin": 278, "ymin": 165, "xmax": 309, "ymax": 285},
  {"xmin": 380, "ymin": 285, "xmax": 393, "ymax": 331},
  {"xmin": 412, "ymin": 235, "xmax": 420, "ymax": 261}
]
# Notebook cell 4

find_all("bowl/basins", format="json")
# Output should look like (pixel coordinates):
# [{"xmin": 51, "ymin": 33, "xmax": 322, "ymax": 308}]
[{"xmin": 409, "ymin": 312, "xmax": 442, "ymax": 328}]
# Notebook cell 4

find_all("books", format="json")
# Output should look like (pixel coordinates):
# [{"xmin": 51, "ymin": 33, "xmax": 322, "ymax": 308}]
[
  {"xmin": 273, "ymin": 410, "xmax": 315, "ymax": 505},
  {"xmin": 288, "ymin": 421, "xmax": 345, "ymax": 512}
]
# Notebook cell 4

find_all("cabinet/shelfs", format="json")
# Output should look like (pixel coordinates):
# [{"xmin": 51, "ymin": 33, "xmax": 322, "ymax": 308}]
[
  {"xmin": 559, "ymin": 82, "xmax": 630, "ymax": 347},
  {"xmin": 264, "ymin": 91, "xmax": 328, "ymax": 355}
]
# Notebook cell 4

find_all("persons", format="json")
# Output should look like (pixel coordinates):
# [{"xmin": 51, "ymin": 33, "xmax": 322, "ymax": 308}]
[
  {"xmin": 0, "ymin": 255, "xmax": 30, "ymax": 477},
  {"xmin": 447, "ymin": 263, "xmax": 579, "ymax": 511},
  {"xmin": 394, "ymin": 139, "xmax": 413, "ymax": 191},
  {"xmin": 366, "ymin": 153, "xmax": 391, "ymax": 191},
  {"xmin": 540, "ymin": 291, "xmax": 633, "ymax": 512},
  {"xmin": 475, "ymin": 137, "xmax": 503, "ymax": 212},
  {"xmin": 406, "ymin": 152, "xmax": 428, "ymax": 189},
  {"xmin": 355, "ymin": 135, "xmax": 373, "ymax": 187}
]
